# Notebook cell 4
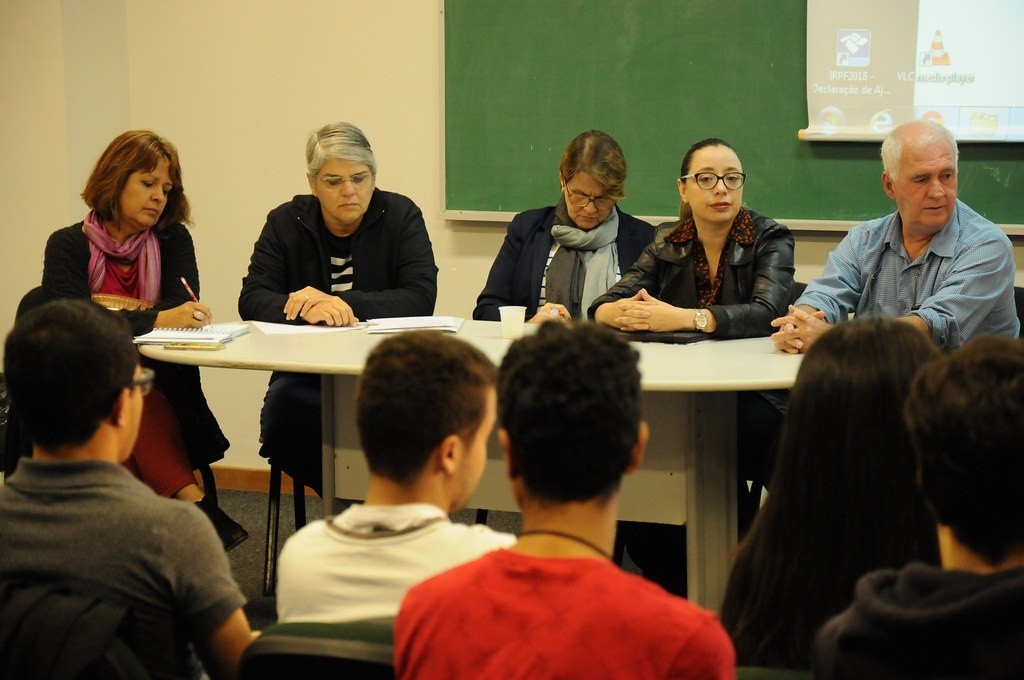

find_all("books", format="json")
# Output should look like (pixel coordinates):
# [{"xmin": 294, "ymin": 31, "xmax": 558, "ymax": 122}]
[{"xmin": 132, "ymin": 324, "xmax": 247, "ymax": 344}]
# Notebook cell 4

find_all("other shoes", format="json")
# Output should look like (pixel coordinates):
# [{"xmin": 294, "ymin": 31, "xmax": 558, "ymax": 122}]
[{"xmin": 193, "ymin": 495, "xmax": 249, "ymax": 552}]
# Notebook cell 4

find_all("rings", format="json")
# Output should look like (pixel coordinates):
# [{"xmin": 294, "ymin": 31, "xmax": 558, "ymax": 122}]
[
  {"xmin": 193, "ymin": 310, "xmax": 204, "ymax": 320},
  {"xmin": 304, "ymin": 294, "xmax": 309, "ymax": 300}
]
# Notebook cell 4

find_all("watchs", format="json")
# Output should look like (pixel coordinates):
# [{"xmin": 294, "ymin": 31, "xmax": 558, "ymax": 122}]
[{"xmin": 693, "ymin": 309, "xmax": 708, "ymax": 332}]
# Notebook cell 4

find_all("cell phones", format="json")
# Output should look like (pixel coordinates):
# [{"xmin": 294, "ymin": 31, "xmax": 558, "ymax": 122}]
[{"xmin": 163, "ymin": 342, "xmax": 225, "ymax": 351}]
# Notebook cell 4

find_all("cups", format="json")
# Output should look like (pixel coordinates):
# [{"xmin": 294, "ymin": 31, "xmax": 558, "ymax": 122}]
[{"xmin": 499, "ymin": 306, "xmax": 527, "ymax": 340}]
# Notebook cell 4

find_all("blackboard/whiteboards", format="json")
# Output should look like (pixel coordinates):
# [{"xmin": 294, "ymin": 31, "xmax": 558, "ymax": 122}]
[{"xmin": 435, "ymin": 0, "xmax": 1024, "ymax": 233}]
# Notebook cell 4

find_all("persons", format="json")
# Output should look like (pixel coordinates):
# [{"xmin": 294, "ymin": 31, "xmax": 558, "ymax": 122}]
[
  {"xmin": 814, "ymin": 338, "xmax": 1024, "ymax": 680},
  {"xmin": 394, "ymin": 320, "xmax": 735, "ymax": 680},
  {"xmin": 239, "ymin": 122, "xmax": 438, "ymax": 498},
  {"xmin": 41, "ymin": 130, "xmax": 249, "ymax": 552},
  {"xmin": 772, "ymin": 121, "xmax": 1021, "ymax": 353},
  {"xmin": 472, "ymin": 132, "xmax": 655, "ymax": 330},
  {"xmin": 0, "ymin": 302, "xmax": 260, "ymax": 680},
  {"xmin": 722, "ymin": 319, "xmax": 943, "ymax": 680},
  {"xmin": 587, "ymin": 138, "xmax": 793, "ymax": 596},
  {"xmin": 275, "ymin": 330, "xmax": 515, "ymax": 622}
]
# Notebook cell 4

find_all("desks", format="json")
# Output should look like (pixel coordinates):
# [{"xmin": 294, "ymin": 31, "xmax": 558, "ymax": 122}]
[{"xmin": 139, "ymin": 322, "xmax": 805, "ymax": 618}]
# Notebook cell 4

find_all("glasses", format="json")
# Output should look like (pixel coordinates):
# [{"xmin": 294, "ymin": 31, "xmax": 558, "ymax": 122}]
[
  {"xmin": 565, "ymin": 180, "xmax": 618, "ymax": 210},
  {"xmin": 312, "ymin": 171, "xmax": 374, "ymax": 189},
  {"xmin": 130, "ymin": 367, "xmax": 154, "ymax": 395},
  {"xmin": 682, "ymin": 171, "xmax": 746, "ymax": 190}
]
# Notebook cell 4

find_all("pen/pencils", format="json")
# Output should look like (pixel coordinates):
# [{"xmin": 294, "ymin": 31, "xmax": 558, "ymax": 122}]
[{"xmin": 180, "ymin": 277, "xmax": 216, "ymax": 329}]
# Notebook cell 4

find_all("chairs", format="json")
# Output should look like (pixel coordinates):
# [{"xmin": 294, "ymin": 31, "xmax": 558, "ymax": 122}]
[
  {"xmin": 233, "ymin": 621, "xmax": 396, "ymax": 680},
  {"xmin": 0, "ymin": 579, "xmax": 158, "ymax": 680}
]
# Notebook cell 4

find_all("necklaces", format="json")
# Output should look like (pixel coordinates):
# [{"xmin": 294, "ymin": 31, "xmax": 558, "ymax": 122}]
[{"xmin": 517, "ymin": 528, "xmax": 609, "ymax": 557}]
